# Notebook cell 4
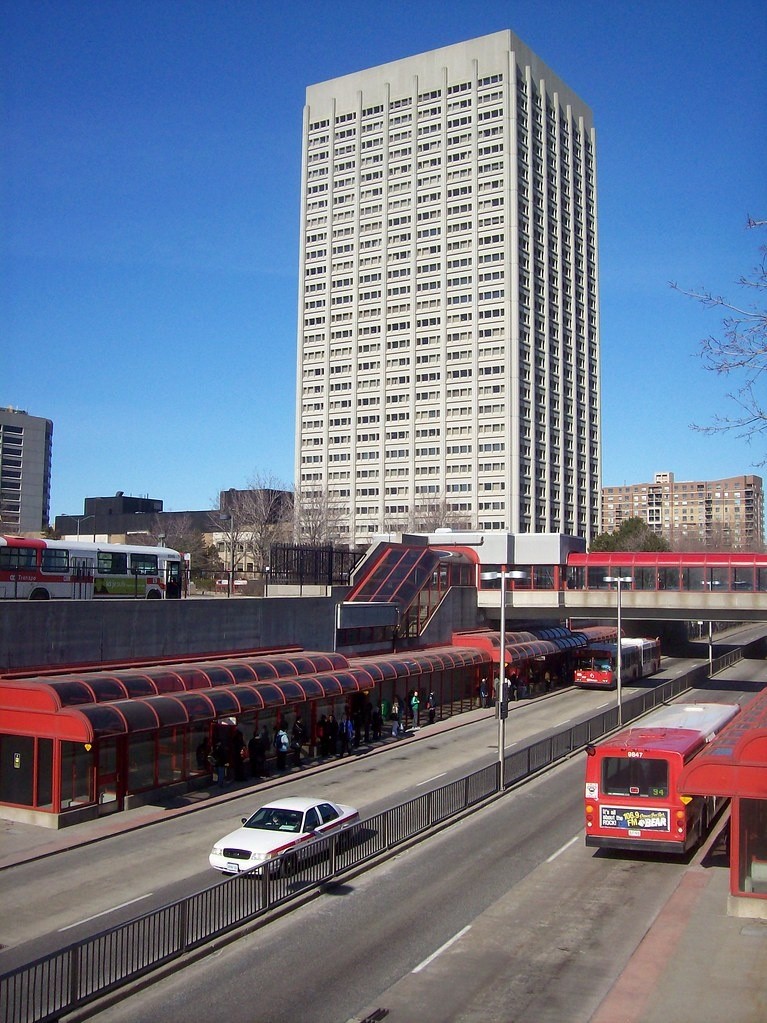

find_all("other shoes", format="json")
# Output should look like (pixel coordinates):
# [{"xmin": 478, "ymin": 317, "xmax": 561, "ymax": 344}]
[{"xmin": 412, "ymin": 727, "xmax": 420, "ymax": 729}]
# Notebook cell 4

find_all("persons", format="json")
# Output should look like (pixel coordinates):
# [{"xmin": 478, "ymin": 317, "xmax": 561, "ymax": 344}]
[
  {"xmin": 480, "ymin": 660, "xmax": 573, "ymax": 709},
  {"xmin": 209, "ymin": 688, "xmax": 436, "ymax": 790},
  {"xmin": 269, "ymin": 814, "xmax": 281, "ymax": 825},
  {"xmin": 567, "ymin": 576, "xmax": 574, "ymax": 588}
]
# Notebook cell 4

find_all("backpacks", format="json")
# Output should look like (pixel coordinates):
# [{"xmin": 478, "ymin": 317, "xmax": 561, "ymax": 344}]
[{"xmin": 273, "ymin": 734, "xmax": 287, "ymax": 749}]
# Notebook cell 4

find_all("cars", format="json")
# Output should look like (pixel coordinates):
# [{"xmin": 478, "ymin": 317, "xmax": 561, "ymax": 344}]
[{"xmin": 209, "ymin": 797, "xmax": 360, "ymax": 879}]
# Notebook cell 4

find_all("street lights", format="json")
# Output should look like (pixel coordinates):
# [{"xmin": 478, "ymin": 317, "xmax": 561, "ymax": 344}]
[
  {"xmin": 220, "ymin": 514, "xmax": 234, "ymax": 593},
  {"xmin": 158, "ymin": 534, "xmax": 166, "ymax": 547},
  {"xmin": 480, "ymin": 571, "xmax": 529, "ymax": 787},
  {"xmin": 602, "ymin": 576, "xmax": 633, "ymax": 726},
  {"xmin": 701, "ymin": 580, "xmax": 722, "ymax": 675},
  {"xmin": 61, "ymin": 514, "xmax": 95, "ymax": 541}
]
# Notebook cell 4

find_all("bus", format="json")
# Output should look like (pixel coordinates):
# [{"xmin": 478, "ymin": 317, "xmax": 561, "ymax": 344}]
[
  {"xmin": 136, "ymin": 567, "xmax": 180, "ymax": 582},
  {"xmin": 573, "ymin": 637, "xmax": 663, "ymax": 690},
  {"xmin": 585, "ymin": 699, "xmax": 741, "ymax": 854},
  {"xmin": 0, "ymin": 534, "xmax": 186, "ymax": 600}
]
